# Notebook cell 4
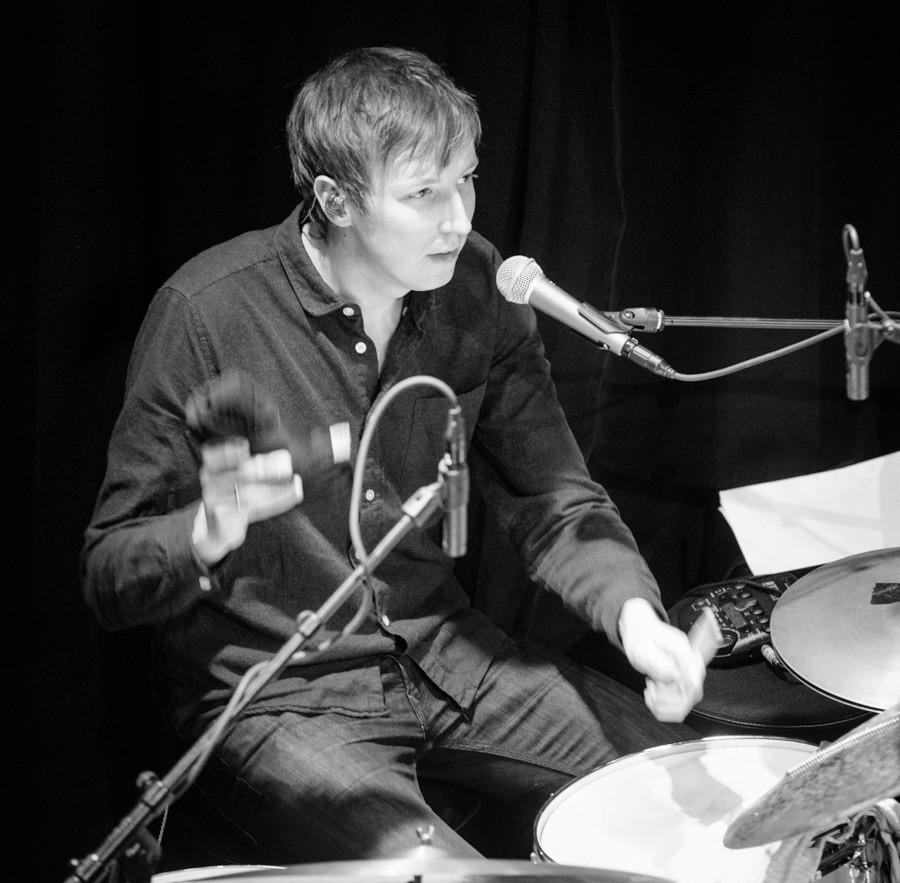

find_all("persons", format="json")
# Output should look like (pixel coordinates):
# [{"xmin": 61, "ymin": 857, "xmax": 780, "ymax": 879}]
[{"xmin": 78, "ymin": 46, "xmax": 706, "ymax": 863}]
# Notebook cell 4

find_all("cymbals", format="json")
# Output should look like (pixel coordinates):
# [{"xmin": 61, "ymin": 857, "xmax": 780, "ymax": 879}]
[
  {"xmin": 723, "ymin": 701, "xmax": 900, "ymax": 849},
  {"xmin": 769, "ymin": 546, "xmax": 900, "ymax": 714},
  {"xmin": 168, "ymin": 846, "xmax": 673, "ymax": 883}
]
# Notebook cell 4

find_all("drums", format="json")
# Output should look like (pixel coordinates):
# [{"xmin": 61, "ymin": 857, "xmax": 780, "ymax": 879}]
[
  {"xmin": 665, "ymin": 576, "xmax": 875, "ymax": 745},
  {"xmin": 532, "ymin": 735, "xmax": 820, "ymax": 883}
]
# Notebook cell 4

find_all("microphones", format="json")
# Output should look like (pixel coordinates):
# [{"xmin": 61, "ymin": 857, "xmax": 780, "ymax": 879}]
[
  {"xmin": 496, "ymin": 253, "xmax": 674, "ymax": 378},
  {"xmin": 440, "ymin": 408, "xmax": 468, "ymax": 557},
  {"xmin": 846, "ymin": 248, "xmax": 870, "ymax": 401}
]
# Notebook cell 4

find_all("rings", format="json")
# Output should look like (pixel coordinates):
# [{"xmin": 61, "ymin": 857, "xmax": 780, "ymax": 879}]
[{"xmin": 233, "ymin": 484, "xmax": 241, "ymax": 507}]
